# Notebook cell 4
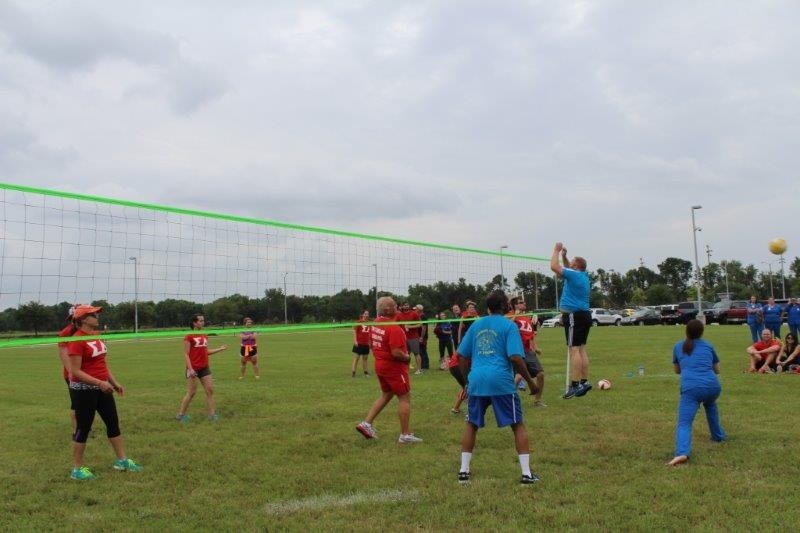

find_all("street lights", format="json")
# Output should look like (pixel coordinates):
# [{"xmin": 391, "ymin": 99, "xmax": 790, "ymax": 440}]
[
  {"xmin": 690, "ymin": 205, "xmax": 706, "ymax": 326},
  {"xmin": 283, "ymin": 272, "xmax": 288, "ymax": 325},
  {"xmin": 761, "ymin": 261, "xmax": 775, "ymax": 299},
  {"xmin": 129, "ymin": 255, "xmax": 138, "ymax": 333},
  {"xmin": 500, "ymin": 245, "xmax": 508, "ymax": 290},
  {"xmin": 372, "ymin": 264, "xmax": 378, "ymax": 317}
]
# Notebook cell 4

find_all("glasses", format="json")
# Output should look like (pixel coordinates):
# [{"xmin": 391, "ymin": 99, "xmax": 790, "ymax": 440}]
[
  {"xmin": 787, "ymin": 337, "xmax": 794, "ymax": 340},
  {"xmin": 518, "ymin": 301, "xmax": 525, "ymax": 304},
  {"xmin": 87, "ymin": 314, "xmax": 101, "ymax": 318}
]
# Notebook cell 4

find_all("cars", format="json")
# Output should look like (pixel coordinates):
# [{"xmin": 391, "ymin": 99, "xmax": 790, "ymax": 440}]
[{"xmin": 542, "ymin": 313, "xmax": 564, "ymax": 328}]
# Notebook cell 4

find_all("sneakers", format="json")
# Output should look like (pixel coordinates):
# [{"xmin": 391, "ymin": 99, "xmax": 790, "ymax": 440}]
[
  {"xmin": 458, "ymin": 472, "xmax": 470, "ymax": 484},
  {"xmin": 113, "ymin": 457, "xmax": 139, "ymax": 471},
  {"xmin": 176, "ymin": 414, "xmax": 189, "ymax": 420},
  {"xmin": 520, "ymin": 473, "xmax": 539, "ymax": 483},
  {"xmin": 355, "ymin": 421, "xmax": 377, "ymax": 438},
  {"xmin": 745, "ymin": 367, "xmax": 766, "ymax": 374},
  {"xmin": 563, "ymin": 384, "xmax": 583, "ymax": 399},
  {"xmin": 239, "ymin": 376, "xmax": 245, "ymax": 379},
  {"xmin": 208, "ymin": 414, "xmax": 218, "ymax": 421},
  {"xmin": 575, "ymin": 380, "xmax": 593, "ymax": 396},
  {"xmin": 352, "ymin": 373, "xmax": 356, "ymax": 378},
  {"xmin": 398, "ymin": 432, "xmax": 422, "ymax": 443},
  {"xmin": 363, "ymin": 373, "xmax": 370, "ymax": 377},
  {"xmin": 414, "ymin": 369, "xmax": 423, "ymax": 374},
  {"xmin": 71, "ymin": 465, "xmax": 95, "ymax": 479},
  {"xmin": 534, "ymin": 401, "xmax": 548, "ymax": 407}
]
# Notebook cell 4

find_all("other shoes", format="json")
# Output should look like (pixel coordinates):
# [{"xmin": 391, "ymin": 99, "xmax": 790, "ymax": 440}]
[{"xmin": 451, "ymin": 408, "xmax": 460, "ymax": 414}]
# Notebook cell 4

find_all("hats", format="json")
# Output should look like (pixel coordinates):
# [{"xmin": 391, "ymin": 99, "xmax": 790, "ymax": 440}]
[
  {"xmin": 74, "ymin": 305, "xmax": 102, "ymax": 319},
  {"xmin": 412, "ymin": 304, "xmax": 423, "ymax": 310},
  {"xmin": 65, "ymin": 307, "xmax": 74, "ymax": 321}
]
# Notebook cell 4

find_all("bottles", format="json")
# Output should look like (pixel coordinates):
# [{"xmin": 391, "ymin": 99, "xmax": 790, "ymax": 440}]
[
  {"xmin": 639, "ymin": 364, "xmax": 645, "ymax": 376},
  {"xmin": 777, "ymin": 363, "xmax": 782, "ymax": 373}
]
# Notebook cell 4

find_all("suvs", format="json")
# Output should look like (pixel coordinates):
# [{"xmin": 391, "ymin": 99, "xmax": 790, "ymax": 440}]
[{"xmin": 589, "ymin": 299, "xmax": 789, "ymax": 327}]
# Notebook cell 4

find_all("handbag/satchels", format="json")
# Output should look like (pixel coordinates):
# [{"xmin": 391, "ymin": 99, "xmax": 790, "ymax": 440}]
[{"xmin": 434, "ymin": 326, "xmax": 442, "ymax": 337}]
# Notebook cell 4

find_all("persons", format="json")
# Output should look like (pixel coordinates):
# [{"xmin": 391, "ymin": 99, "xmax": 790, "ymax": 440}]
[
  {"xmin": 237, "ymin": 317, "xmax": 261, "ymax": 381},
  {"xmin": 174, "ymin": 314, "xmax": 229, "ymax": 423},
  {"xmin": 349, "ymin": 297, "xmax": 547, "ymax": 444},
  {"xmin": 667, "ymin": 319, "xmax": 727, "ymax": 465},
  {"xmin": 746, "ymin": 295, "xmax": 800, "ymax": 375},
  {"xmin": 551, "ymin": 242, "xmax": 593, "ymax": 399},
  {"xmin": 58, "ymin": 304, "xmax": 142, "ymax": 479},
  {"xmin": 455, "ymin": 291, "xmax": 540, "ymax": 486}
]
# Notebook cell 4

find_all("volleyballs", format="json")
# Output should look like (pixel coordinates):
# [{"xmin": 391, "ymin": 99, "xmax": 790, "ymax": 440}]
[
  {"xmin": 597, "ymin": 379, "xmax": 611, "ymax": 390},
  {"xmin": 768, "ymin": 237, "xmax": 787, "ymax": 254}
]
